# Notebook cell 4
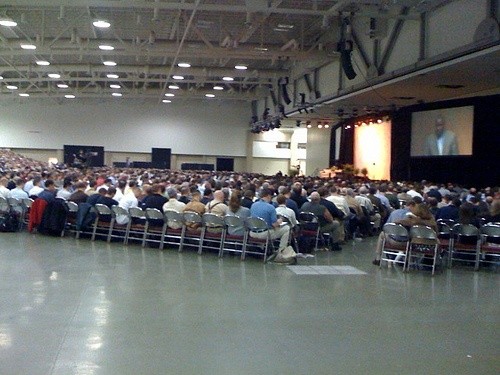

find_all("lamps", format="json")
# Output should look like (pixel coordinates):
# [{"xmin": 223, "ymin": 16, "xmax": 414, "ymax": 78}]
[
  {"xmin": 250, "ymin": 119, "xmax": 281, "ymax": 135},
  {"xmin": 295, "ymin": 108, "xmax": 392, "ymax": 130}
]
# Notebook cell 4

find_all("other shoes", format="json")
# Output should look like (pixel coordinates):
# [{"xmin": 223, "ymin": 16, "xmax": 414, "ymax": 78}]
[
  {"xmin": 372, "ymin": 259, "xmax": 380, "ymax": 265},
  {"xmin": 331, "ymin": 245, "xmax": 343, "ymax": 251}
]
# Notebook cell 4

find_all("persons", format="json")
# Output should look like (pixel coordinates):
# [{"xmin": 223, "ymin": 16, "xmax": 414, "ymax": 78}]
[
  {"xmin": 1, "ymin": 147, "xmax": 500, "ymax": 273},
  {"xmin": 422, "ymin": 114, "xmax": 459, "ymax": 155}
]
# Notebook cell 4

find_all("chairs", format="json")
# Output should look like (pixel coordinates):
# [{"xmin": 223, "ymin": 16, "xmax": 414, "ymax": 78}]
[
  {"xmin": 378, "ymin": 216, "xmax": 500, "ymax": 278},
  {"xmin": 0, "ymin": 194, "xmax": 331, "ymax": 263}
]
